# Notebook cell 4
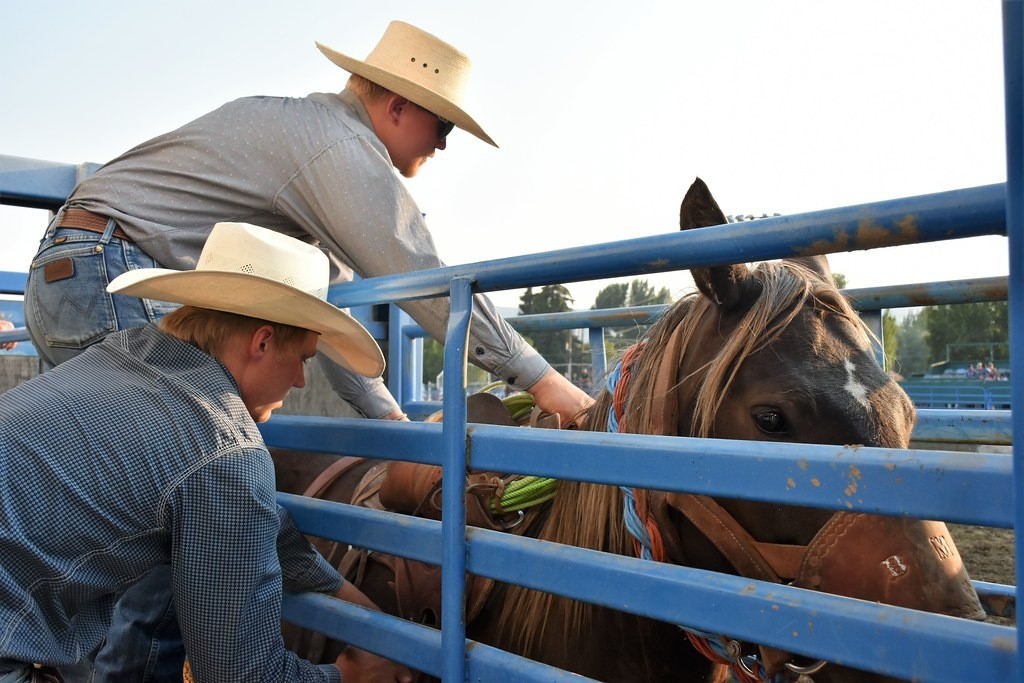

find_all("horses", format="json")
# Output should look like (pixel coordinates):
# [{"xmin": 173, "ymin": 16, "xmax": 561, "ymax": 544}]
[{"xmin": 268, "ymin": 174, "xmax": 990, "ymax": 683}]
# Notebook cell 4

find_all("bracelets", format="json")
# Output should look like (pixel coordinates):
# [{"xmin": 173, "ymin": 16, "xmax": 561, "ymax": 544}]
[{"xmin": 395, "ymin": 414, "xmax": 407, "ymax": 420}]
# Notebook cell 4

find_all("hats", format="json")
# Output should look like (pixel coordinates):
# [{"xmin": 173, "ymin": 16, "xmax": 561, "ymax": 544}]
[
  {"xmin": 315, "ymin": 20, "xmax": 500, "ymax": 149},
  {"xmin": 106, "ymin": 222, "xmax": 386, "ymax": 379}
]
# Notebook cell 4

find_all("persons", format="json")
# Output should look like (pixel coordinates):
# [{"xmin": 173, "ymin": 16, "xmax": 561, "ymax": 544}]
[
  {"xmin": 23, "ymin": 20, "xmax": 597, "ymax": 429},
  {"xmin": 965, "ymin": 361, "xmax": 1010, "ymax": 410},
  {"xmin": 0, "ymin": 222, "xmax": 412, "ymax": 683},
  {"xmin": 0, "ymin": 320, "xmax": 17, "ymax": 351}
]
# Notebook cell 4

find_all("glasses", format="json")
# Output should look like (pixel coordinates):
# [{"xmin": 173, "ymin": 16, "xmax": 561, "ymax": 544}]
[{"xmin": 410, "ymin": 102, "xmax": 455, "ymax": 140}]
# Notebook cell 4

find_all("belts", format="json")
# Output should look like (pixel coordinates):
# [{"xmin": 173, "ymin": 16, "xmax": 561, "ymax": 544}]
[{"xmin": 47, "ymin": 209, "xmax": 131, "ymax": 242}]
